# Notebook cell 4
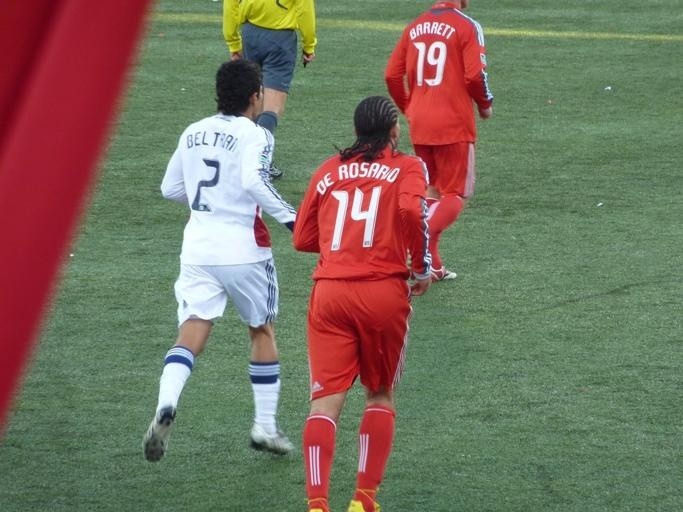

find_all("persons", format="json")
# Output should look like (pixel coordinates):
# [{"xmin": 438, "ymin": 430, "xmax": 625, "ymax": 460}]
[
  {"xmin": 222, "ymin": 1, "xmax": 318, "ymax": 180},
  {"xmin": 294, "ymin": 95, "xmax": 433, "ymax": 510},
  {"xmin": 385, "ymin": 1, "xmax": 495, "ymax": 280},
  {"xmin": 138, "ymin": 58, "xmax": 296, "ymax": 461}
]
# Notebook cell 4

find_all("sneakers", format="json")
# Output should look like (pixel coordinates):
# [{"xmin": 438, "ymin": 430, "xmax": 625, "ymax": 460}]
[
  {"xmin": 429, "ymin": 267, "xmax": 458, "ymax": 283},
  {"xmin": 244, "ymin": 428, "xmax": 298, "ymax": 456},
  {"xmin": 344, "ymin": 497, "xmax": 386, "ymax": 512},
  {"xmin": 141, "ymin": 403, "xmax": 176, "ymax": 466},
  {"xmin": 270, "ymin": 162, "xmax": 285, "ymax": 179}
]
